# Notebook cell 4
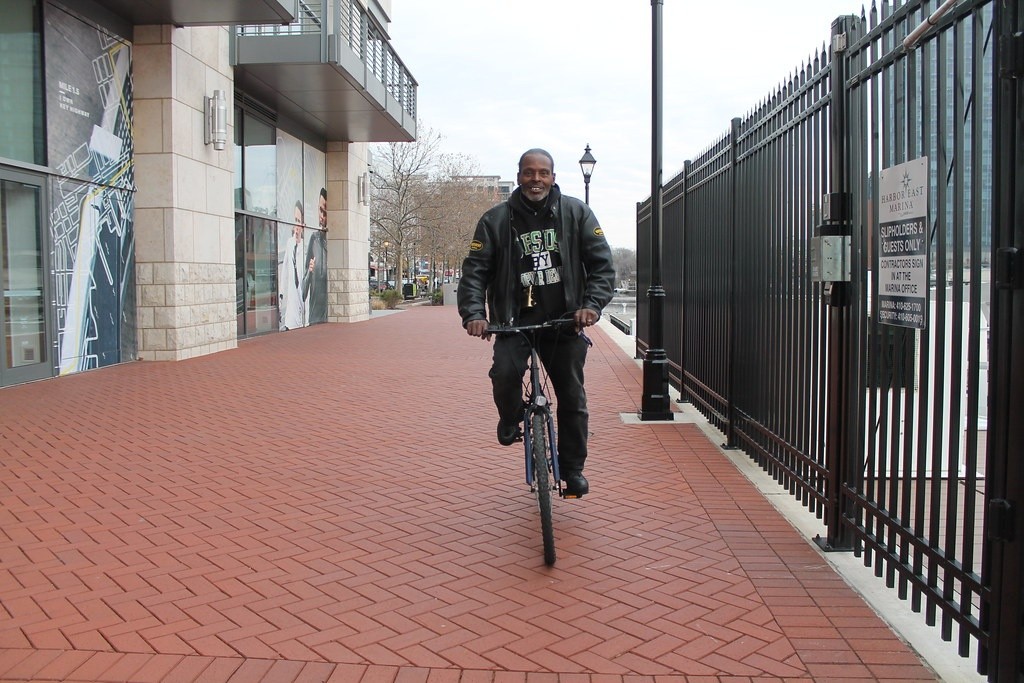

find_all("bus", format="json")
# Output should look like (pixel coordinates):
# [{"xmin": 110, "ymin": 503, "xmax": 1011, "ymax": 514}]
[{"xmin": 370, "ymin": 261, "xmax": 387, "ymax": 291}]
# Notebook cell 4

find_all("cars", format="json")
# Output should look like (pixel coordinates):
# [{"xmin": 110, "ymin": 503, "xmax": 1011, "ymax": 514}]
[{"xmin": 387, "ymin": 269, "xmax": 463, "ymax": 300}]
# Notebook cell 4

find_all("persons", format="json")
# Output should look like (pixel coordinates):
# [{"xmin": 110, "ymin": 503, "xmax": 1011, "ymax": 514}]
[
  {"xmin": 278, "ymin": 199, "xmax": 307, "ymax": 332},
  {"xmin": 457, "ymin": 148, "xmax": 615, "ymax": 494},
  {"xmin": 300, "ymin": 187, "xmax": 329, "ymax": 325}
]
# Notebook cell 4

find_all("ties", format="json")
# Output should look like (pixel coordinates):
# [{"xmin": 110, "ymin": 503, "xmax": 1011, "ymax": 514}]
[{"xmin": 292, "ymin": 243, "xmax": 299, "ymax": 289}]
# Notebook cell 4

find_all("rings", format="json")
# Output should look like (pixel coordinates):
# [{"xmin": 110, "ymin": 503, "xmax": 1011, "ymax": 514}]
[{"xmin": 587, "ymin": 319, "xmax": 593, "ymax": 323}]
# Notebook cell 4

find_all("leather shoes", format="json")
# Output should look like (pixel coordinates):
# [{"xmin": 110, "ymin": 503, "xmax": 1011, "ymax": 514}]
[
  {"xmin": 559, "ymin": 469, "xmax": 589, "ymax": 495},
  {"xmin": 496, "ymin": 412, "xmax": 524, "ymax": 446}
]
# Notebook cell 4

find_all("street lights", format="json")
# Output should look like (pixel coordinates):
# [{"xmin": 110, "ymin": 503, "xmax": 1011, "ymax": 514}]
[
  {"xmin": 384, "ymin": 241, "xmax": 389, "ymax": 289},
  {"xmin": 578, "ymin": 143, "xmax": 597, "ymax": 207}
]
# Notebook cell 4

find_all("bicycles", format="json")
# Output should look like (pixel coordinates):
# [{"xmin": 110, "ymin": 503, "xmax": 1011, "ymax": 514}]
[{"xmin": 485, "ymin": 319, "xmax": 582, "ymax": 566}]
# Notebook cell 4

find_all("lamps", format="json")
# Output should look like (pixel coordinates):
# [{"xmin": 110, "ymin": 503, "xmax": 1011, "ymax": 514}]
[
  {"xmin": 358, "ymin": 172, "xmax": 369, "ymax": 205},
  {"xmin": 204, "ymin": 90, "xmax": 227, "ymax": 150}
]
